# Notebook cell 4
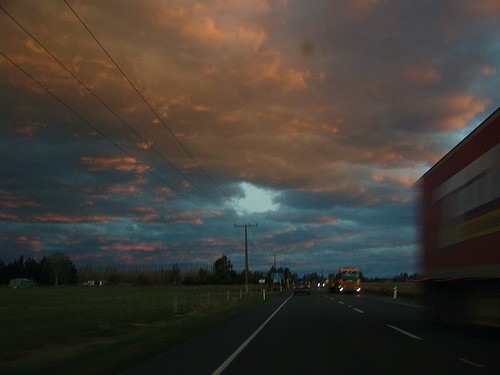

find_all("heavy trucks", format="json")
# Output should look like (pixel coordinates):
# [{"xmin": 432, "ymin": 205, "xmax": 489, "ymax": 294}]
[{"xmin": 328, "ymin": 265, "xmax": 361, "ymax": 295}]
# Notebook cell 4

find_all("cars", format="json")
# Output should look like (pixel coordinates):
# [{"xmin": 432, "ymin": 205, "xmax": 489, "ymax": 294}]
[
  {"xmin": 317, "ymin": 282, "xmax": 325, "ymax": 288},
  {"xmin": 292, "ymin": 282, "xmax": 311, "ymax": 294}
]
